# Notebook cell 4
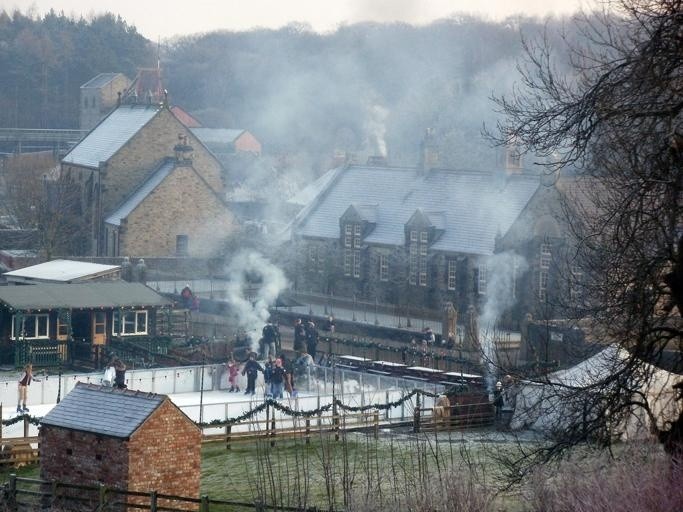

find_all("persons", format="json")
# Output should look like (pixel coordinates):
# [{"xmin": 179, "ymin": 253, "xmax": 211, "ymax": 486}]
[
  {"xmin": 180, "ymin": 282, "xmax": 192, "ymax": 310},
  {"xmin": 255, "ymin": 315, "xmax": 320, "ymax": 400},
  {"xmin": 241, "ymin": 353, "xmax": 265, "ymax": 395},
  {"xmin": 101, "ymin": 359, "xmax": 116, "ymax": 389},
  {"xmin": 16, "ymin": 363, "xmax": 43, "ymax": 411},
  {"xmin": 115, "ymin": 360, "xmax": 126, "ymax": 384},
  {"xmin": 224, "ymin": 359, "xmax": 240, "ymax": 393},
  {"xmin": 191, "ymin": 296, "xmax": 201, "ymax": 313},
  {"xmin": 324, "ymin": 313, "xmax": 336, "ymax": 337}
]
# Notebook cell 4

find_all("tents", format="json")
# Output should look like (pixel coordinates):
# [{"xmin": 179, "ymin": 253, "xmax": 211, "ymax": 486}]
[{"xmin": 510, "ymin": 340, "xmax": 683, "ymax": 443}]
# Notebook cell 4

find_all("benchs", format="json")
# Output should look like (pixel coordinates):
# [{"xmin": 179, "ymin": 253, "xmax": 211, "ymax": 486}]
[{"xmin": 337, "ymin": 354, "xmax": 482, "ymax": 393}]
